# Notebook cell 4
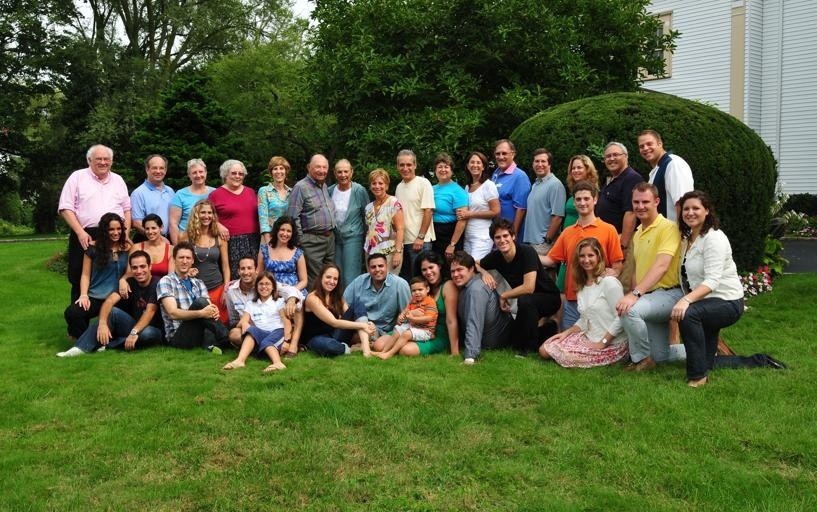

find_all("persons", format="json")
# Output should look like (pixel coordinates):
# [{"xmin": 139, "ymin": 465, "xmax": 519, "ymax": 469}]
[
  {"xmin": 58, "ymin": 139, "xmax": 630, "ymax": 373},
  {"xmin": 617, "ymin": 181, "xmax": 728, "ymax": 373},
  {"xmin": 637, "ymin": 131, "xmax": 695, "ymax": 344},
  {"xmin": 595, "ymin": 142, "xmax": 648, "ymax": 296},
  {"xmin": 669, "ymin": 190, "xmax": 786, "ymax": 387}
]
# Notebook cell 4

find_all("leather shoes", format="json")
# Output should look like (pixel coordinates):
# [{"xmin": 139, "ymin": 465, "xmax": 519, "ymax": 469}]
[
  {"xmin": 264, "ymin": 365, "xmax": 280, "ymax": 373},
  {"xmin": 765, "ymin": 355, "xmax": 785, "ymax": 371}
]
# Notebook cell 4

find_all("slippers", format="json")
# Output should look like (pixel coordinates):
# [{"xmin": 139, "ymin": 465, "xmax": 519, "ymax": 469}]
[{"xmin": 220, "ymin": 362, "xmax": 236, "ymax": 372}]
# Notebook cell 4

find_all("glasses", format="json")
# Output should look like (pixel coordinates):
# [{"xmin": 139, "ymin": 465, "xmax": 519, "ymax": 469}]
[{"xmin": 604, "ymin": 152, "xmax": 626, "ymax": 159}]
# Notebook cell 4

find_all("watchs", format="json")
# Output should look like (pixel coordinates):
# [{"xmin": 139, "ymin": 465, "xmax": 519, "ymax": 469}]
[{"xmin": 631, "ymin": 289, "xmax": 641, "ymax": 298}]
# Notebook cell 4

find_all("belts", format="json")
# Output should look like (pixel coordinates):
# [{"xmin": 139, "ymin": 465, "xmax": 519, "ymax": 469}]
[{"xmin": 304, "ymin": 230, "xmax": 334, "ymax": 236}]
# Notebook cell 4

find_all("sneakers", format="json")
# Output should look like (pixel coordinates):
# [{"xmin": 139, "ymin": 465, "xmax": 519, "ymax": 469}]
[
  {"xmin": 56, "ymin": 347, "xmax": 84, "ymax": 357},
  {"xmin": 624, "ymin": 359, "xmax": 656, "ymax": 372}
]
